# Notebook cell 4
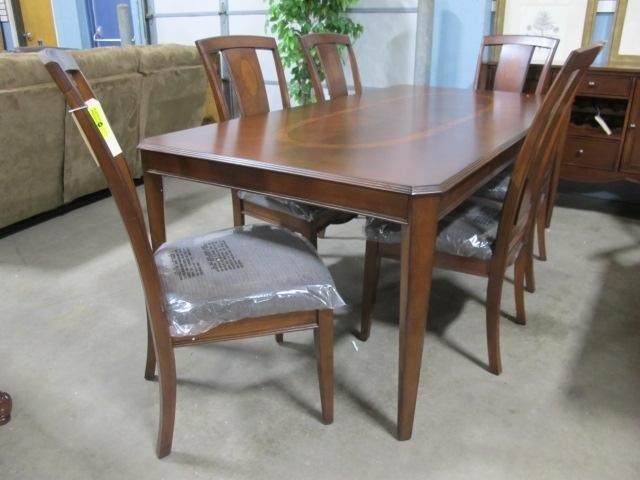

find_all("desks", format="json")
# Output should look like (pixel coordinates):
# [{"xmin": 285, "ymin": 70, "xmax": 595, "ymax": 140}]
[{"xmin": 137, "ymin": 85, "xmax": 546, "ymax": 441}]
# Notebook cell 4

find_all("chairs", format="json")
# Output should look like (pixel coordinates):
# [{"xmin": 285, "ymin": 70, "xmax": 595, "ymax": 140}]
[
  {"xmin": 37, "ymin": 48, "xmax": 336, "ymax": 460},
  {"xmin": 299, "ymin": 33, "xmax": 363, "ymax": 103},
  {"xmin": 473, "ymin": 34, "xmax": 561, "ymax": 94},
  {"xmin": 195, "ymin": 35, "xmax": 358, "ymax": 251},
  {"xmin": 473, "ymin": 39, "xmax": 607, "ymax": 292},
  {"xmin": 360, "ymin": 42, "xmax": 603, "ymax": 375}
]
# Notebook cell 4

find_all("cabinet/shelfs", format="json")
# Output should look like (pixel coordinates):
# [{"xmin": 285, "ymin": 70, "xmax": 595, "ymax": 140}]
[{"xmin": 478, "ymin": 60, "xmax": 639, "ymax": 185}]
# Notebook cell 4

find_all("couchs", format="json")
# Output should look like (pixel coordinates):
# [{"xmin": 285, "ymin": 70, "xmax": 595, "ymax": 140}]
[{"xmin": 1, "ymin": 43, "xmax": 210, "ymax": 229}]
